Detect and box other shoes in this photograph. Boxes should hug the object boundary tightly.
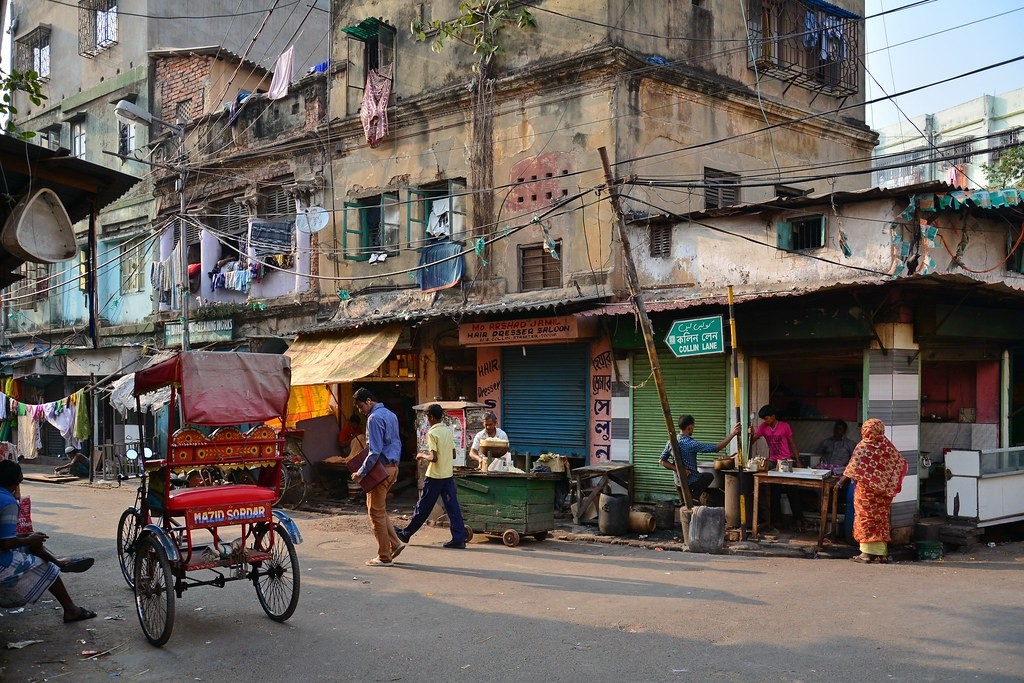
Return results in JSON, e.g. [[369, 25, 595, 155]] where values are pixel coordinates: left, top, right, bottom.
[[442, 539, 467, 548], [393, 524, 409, 543]]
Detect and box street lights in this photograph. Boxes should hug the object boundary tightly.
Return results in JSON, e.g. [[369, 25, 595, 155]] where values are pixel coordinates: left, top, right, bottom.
[[110, 100, 189, 353]]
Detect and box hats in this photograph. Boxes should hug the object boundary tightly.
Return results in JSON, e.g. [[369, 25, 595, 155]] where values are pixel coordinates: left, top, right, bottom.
[[65, 447, 76, 453]]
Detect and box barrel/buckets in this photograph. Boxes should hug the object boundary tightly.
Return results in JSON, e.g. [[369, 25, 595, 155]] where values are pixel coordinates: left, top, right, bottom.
[[628, 512, 656, 534], [598, 492, 629, 535], [348, 480, 365, 500], [915, 540, 944, 560], [655, 500, 675, 530]]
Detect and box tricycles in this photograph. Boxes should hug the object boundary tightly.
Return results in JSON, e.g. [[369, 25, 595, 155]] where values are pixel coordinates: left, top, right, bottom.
[[181, 428, 310, 511], [115, 350, 303, 645]]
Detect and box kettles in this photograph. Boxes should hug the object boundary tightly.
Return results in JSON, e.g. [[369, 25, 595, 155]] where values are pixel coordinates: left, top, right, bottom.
[[744, 456, 767, 472]]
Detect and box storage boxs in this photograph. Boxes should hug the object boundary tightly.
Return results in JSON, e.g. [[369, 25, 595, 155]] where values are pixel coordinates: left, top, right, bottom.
[[532, 457, 566, 472]]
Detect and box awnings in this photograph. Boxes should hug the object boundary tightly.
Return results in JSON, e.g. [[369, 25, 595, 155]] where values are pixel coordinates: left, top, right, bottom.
[[283, 325, 402, 387]]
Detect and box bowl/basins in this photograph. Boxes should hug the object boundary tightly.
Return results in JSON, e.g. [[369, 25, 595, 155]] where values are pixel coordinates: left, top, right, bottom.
[[777, 459, 794, 472], [479, 446, 509, 458]]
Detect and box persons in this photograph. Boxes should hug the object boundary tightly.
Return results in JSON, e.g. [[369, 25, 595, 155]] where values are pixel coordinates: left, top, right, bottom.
[[815, 420, 856, 466], [394, 404, 466, 549], [0, 459, 97, 623], [351, 388, 406, 566], [55, 447, 89, 477], [833, 419, 907, 564], [660, 415, 742, 503], [469, 411, 510, 468], [747, 405, 803, 530]]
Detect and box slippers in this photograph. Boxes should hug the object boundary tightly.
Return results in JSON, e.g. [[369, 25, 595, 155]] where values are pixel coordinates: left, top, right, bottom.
[[365, 558, 395, 567], [62, 605, 99, 623], [849, 556, 871, 563], [872, 560, 882, 563], [61, 555, 94, 572], [390, 543, 405, 557]]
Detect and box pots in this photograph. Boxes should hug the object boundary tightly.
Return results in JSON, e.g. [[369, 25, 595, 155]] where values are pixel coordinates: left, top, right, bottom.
[[696, 465, 725, 488], [919, 451, 931, 478], [713, 452, 737, 470]]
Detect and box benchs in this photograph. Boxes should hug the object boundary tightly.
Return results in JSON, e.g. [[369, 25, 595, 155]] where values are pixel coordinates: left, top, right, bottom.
[[143, 425, 288, 512]]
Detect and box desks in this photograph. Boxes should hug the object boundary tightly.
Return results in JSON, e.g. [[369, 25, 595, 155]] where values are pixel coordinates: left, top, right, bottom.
[[752, 471, 838, 550], [570, 460, 635, 525], [312, 461, 349, 485]]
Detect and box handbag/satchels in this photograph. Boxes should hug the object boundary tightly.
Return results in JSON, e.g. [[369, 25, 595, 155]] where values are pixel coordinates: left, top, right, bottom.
[[348, 446, 389, 494]]
